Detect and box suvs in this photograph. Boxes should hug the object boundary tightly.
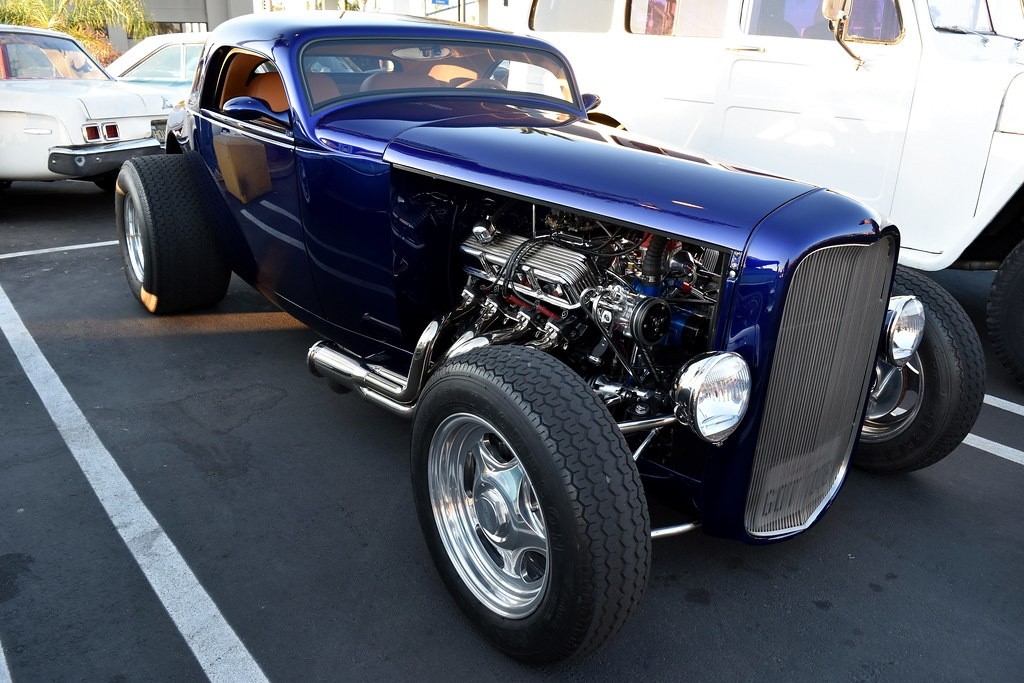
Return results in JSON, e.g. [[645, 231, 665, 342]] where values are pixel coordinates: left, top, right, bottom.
[[495, 0, 1023, 389]]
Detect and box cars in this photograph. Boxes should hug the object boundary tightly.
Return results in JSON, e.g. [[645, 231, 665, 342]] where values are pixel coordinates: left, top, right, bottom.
[[1, 24, 189, 215], [109, 11, 987, 673], [101, 28, 368, 98]]
